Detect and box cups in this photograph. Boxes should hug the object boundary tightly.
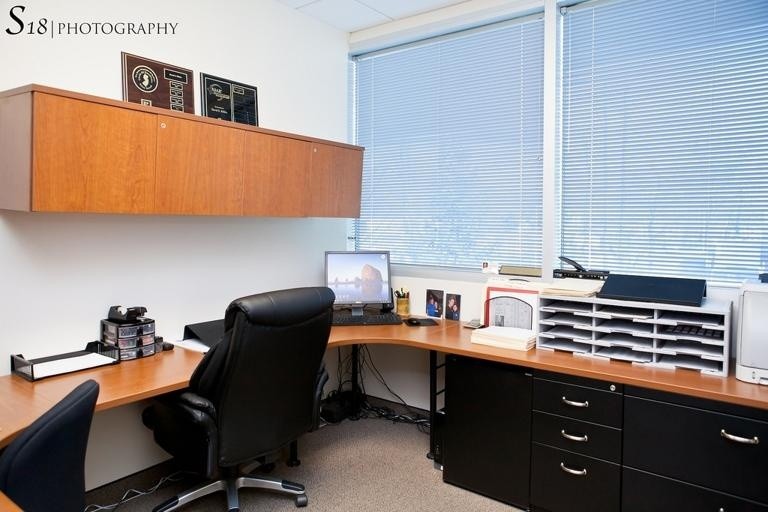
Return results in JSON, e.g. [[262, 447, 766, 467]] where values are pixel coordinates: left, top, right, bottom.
[[396, 298, 409, 314]]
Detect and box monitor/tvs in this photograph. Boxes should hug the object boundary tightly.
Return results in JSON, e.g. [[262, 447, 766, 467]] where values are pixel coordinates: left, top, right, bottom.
[[324, 250, 391, 316]]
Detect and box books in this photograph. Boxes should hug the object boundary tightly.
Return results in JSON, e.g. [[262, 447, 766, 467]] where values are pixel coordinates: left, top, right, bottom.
[[469, 275, 605, 351]]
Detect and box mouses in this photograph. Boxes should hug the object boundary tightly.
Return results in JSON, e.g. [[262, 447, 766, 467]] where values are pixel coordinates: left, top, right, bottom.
[[407, 319, 422, 326]]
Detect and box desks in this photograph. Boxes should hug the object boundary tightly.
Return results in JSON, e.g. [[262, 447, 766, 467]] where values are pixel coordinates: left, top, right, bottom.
[[1, 314, 768, 512]]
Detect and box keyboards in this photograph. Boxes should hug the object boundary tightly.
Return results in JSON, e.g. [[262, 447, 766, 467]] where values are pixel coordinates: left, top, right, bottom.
[[332, 316, 403, 326]]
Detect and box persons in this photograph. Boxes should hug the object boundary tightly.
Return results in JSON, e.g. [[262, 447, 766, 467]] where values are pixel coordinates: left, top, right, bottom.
[[444, 295, 457, 321], [452, 304, 459, 321], [434, 301, 442, 318], [426, 294, 436, 316]]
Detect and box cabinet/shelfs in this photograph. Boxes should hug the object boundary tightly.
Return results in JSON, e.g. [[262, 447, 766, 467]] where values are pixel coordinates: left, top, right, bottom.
[[443, 353, 768, 512], [0, 83, 367, 217], [534, 288, 734, 377]]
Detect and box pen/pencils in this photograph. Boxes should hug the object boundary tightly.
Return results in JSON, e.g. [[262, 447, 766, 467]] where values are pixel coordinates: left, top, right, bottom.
[[394, 287, 409, 298]]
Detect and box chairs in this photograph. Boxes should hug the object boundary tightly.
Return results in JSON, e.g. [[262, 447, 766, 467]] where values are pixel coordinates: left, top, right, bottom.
[[1, 378, 100, 512], [140, 286, 335, 512]]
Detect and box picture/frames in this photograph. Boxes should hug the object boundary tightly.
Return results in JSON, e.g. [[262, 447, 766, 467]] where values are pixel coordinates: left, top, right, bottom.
[[120, 52, 258, 127]]
